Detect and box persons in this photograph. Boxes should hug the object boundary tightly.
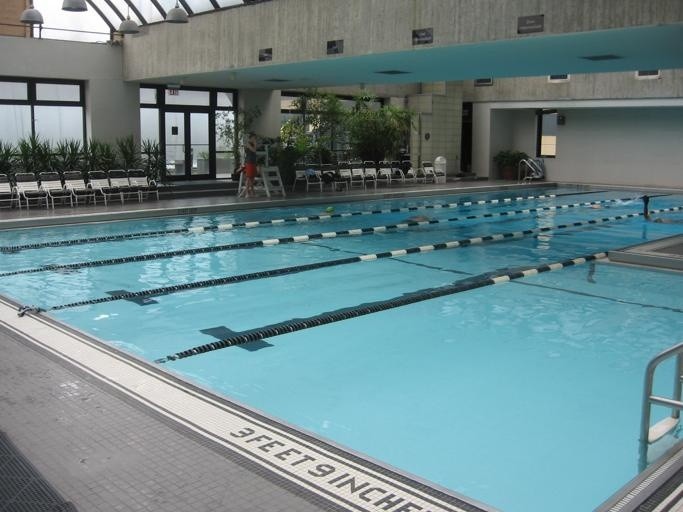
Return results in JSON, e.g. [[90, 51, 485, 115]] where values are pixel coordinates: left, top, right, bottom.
[[245, 134, 259, 198]]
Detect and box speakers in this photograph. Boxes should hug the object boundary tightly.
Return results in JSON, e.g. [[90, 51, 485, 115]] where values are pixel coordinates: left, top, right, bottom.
[[557, 115, 565, 125]]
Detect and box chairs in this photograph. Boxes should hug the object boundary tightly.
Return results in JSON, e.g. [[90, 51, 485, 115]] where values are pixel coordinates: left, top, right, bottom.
[[0, 169, 161, 211], [291, 159, 438, 193]]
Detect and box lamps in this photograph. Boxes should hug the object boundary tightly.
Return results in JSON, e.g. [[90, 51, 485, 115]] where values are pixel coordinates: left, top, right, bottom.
[[18, 1, 189, 34]]
